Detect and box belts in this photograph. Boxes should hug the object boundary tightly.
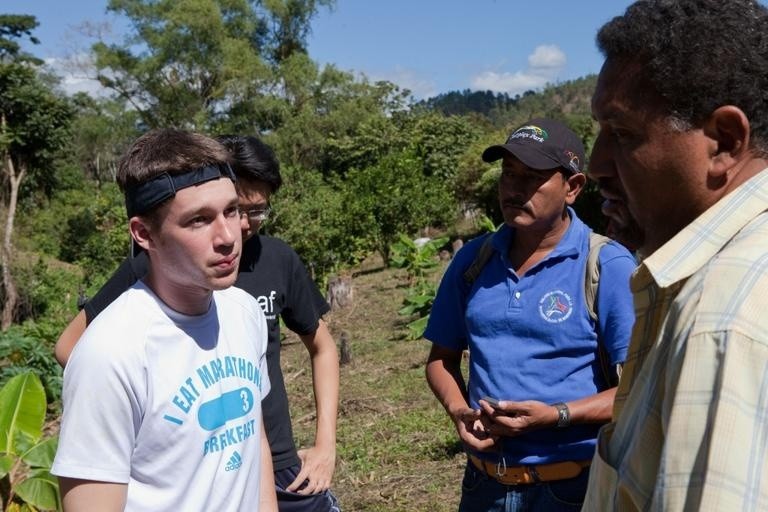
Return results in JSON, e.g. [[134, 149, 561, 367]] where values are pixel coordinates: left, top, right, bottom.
[[467, 454, 592, 485]]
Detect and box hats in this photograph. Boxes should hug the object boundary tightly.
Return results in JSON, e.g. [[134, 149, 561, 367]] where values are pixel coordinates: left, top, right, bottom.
[[482, 116, 586, 176], [126, 158, 237, 260]]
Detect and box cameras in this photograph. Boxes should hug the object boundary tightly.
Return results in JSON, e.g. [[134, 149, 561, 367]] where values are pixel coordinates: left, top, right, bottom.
[[481, 396, 520, 425]]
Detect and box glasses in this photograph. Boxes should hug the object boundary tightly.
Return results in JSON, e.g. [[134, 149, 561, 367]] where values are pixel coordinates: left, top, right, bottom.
[[242, 201, 274, 222]]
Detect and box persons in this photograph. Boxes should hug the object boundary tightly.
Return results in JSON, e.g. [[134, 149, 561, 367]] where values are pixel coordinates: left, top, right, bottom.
[[579, 1, 767, 512], [50, 126, 280, 512], [424, 118, 639, 512], [54, 133, 343, 512]]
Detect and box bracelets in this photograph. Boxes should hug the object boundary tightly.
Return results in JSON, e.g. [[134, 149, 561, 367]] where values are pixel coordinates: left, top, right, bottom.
[[550, 401, 571, 430]]
